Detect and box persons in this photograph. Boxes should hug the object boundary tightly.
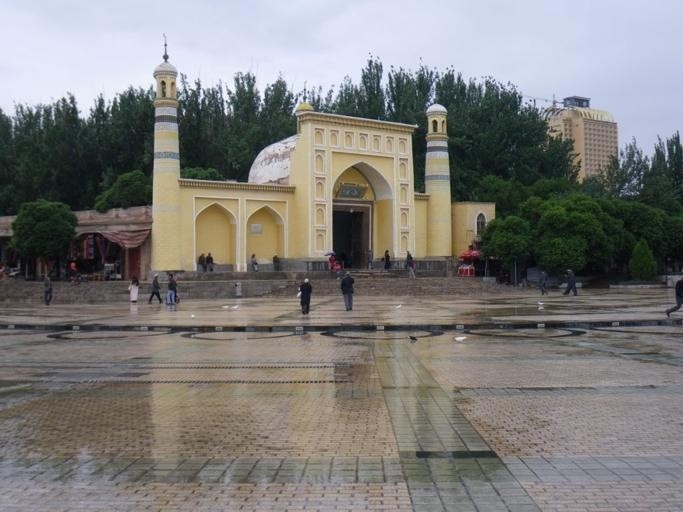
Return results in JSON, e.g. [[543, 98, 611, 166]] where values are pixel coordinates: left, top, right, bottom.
[[537, 269, 548, 295], [299, 278, 311, 314], [328, 254, 336, 272], [196, 253, 205, 273], [127, 275, 139, 303], [407, 257, 415, 280], [332, 261, 343, 279], [166, 273, 176, 305], [43, 274, 52, 306], [560, 268, 577, 295], [664, 276, 682, 318], [340, 271, 354, 311], [148, 273, 162, 304], [383, 249, 391, 270], [205, 253, 212, 272], [272, 253, 280, 271], [250, 254, 257, 272], [404, 250, 412, 269]]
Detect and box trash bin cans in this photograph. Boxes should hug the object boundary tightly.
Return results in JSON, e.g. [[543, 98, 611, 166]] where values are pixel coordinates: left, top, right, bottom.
[[666, 275, 673, 288], [234, 283, 242, 297]]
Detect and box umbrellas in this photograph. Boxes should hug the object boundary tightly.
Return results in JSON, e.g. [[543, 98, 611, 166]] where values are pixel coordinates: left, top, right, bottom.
[[470, 247, 497, 277], [323, 251, 336, 256], [457, 248, 476, 266]]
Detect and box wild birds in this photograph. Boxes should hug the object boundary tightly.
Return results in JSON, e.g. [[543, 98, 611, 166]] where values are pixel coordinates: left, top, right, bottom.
[[222, 305, 229, 309], [191, 314, 195, 319], [396, 304, 402, 309], [537, 300, 545, 305], [409, 334, 418, 343], [537, 306, 544, 311], [232, 305, 239, 310], [452, 336, 468, 343]]
